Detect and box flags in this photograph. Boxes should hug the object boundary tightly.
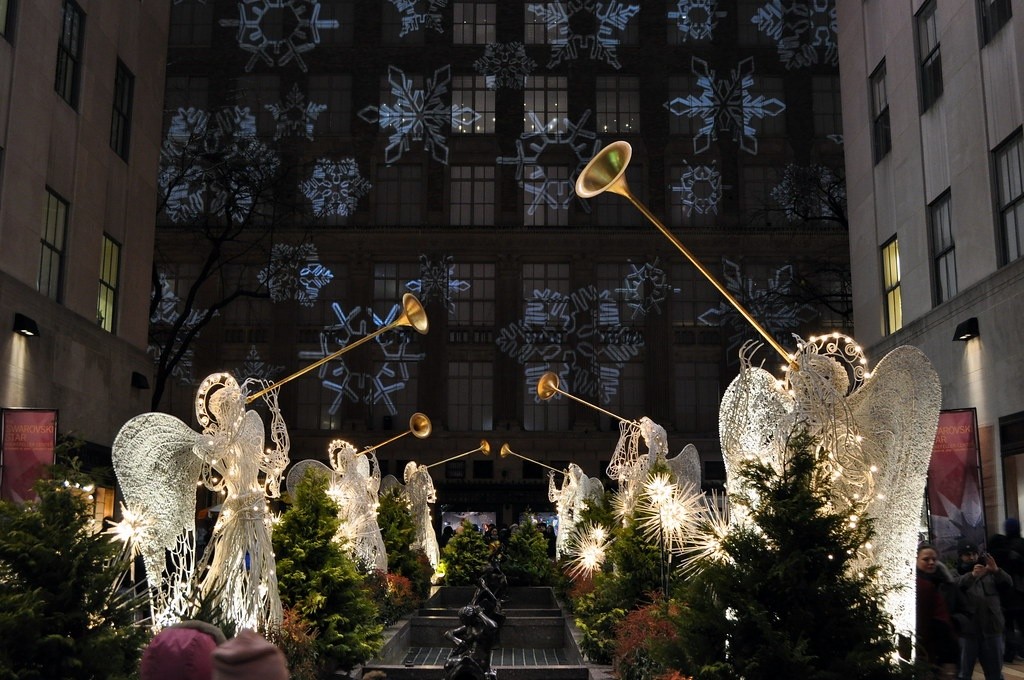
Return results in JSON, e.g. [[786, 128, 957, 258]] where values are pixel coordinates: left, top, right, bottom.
[[2, 410, 57, 513], [926, 412, 987, 561]]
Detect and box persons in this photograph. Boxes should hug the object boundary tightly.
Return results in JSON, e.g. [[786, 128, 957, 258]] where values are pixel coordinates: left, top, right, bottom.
[[916, 518, 1024, 680], [91, 517, 115, 539], [439, 523, 557, 559]]
